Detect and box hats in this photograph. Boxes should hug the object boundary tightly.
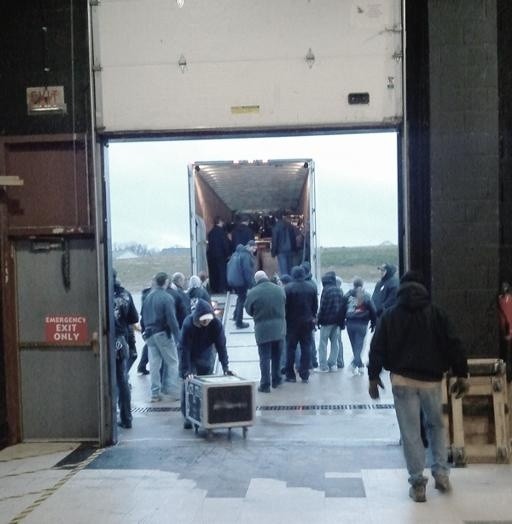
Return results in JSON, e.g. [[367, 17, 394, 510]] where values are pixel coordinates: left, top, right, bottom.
[[247, 240, 257, 247], [241, 214, 249, 221], [276, 209, 282, 219], [379, 264, 386, 271]]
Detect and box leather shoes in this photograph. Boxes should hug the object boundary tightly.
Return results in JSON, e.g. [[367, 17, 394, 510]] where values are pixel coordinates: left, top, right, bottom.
[[258, 387, 269, 392], [183, 417, 193, 429], [137, 369, 150, 375], [272, 378, 282, 387], [236, 322, 249, 329]]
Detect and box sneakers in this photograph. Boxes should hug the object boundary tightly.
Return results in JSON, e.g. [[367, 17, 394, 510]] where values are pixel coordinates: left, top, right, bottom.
[[408, 483, 426, 501], [435, 478, 450, 492], [170, 397, 180, 402], [151, 396, 162, 403], [357, 367, 364, 374], [281, 367, 286, 374], [313, 364, 338, 373], [117, 421, 132, 428], [286, 378, 295, 382], [302, 379, 308, 384], [347, 365, 356, 375]]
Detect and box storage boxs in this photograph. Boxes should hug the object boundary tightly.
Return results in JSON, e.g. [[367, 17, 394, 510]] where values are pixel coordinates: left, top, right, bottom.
[[185, 374, 257, 429]]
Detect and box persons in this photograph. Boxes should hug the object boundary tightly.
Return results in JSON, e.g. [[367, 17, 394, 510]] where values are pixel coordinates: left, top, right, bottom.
[[186, 275, 209, 303], [207, 217, 229, 293], [314, 271, 343, 372], [343, 279, 377, 378], [177, 299, 237, 430], [371, 263, 399, 327], [245, 269, 286, 394], [270, 272, 281, 286], [335, 277, 344, 369], [141, 272, 181, 404], [126, 324, 137, 389], [271, 213, 295, 275], [171, 273, 191, 314], [280, 275, 301, 374], [498, 281, 511, 384], [166, 276, 188, 330], [198, 271, 212, 297], [112, 268, 139, 429], [226, 241, 258, 329], [232, 216, 255, 253], [137, 287, 152, 375], [367, 271, 469, 503], [284, 267, 318, 385], [300, 262, 318, 368]]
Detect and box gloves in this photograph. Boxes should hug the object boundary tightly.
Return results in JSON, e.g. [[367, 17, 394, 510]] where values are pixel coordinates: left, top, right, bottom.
[[271, 249, 276, 256], [450, 376, 470, 399], [368, 376, 383, 400], [184, 371, 195, 380], [225, 368, 239, 376], [368, 324, 375, 333]]
